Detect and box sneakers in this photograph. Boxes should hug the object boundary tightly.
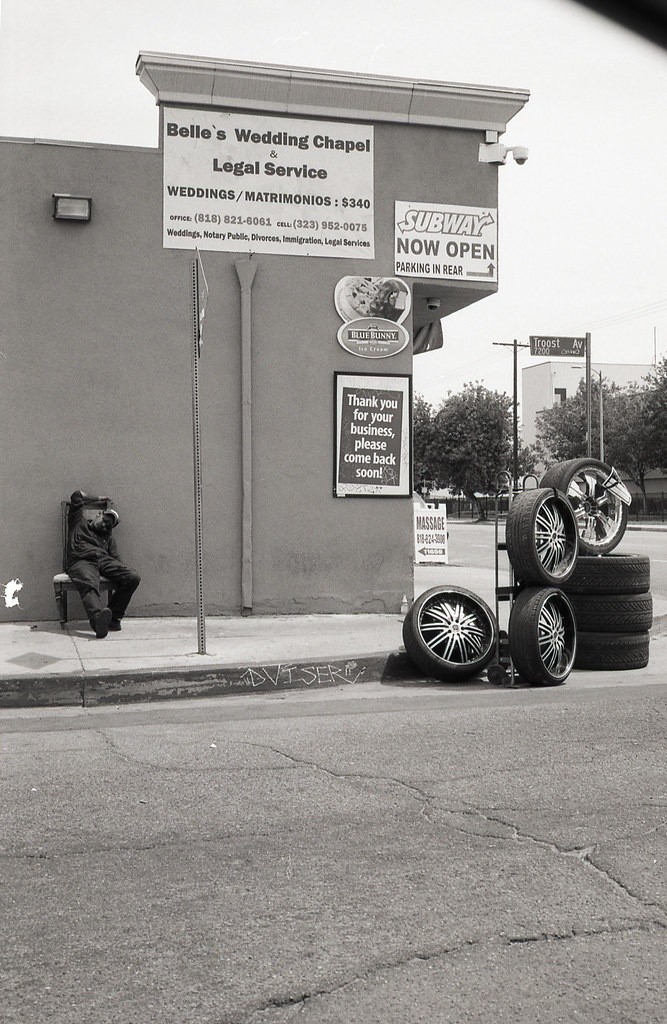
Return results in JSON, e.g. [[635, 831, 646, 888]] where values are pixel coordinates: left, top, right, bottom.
[[91, 608, 121, 638]]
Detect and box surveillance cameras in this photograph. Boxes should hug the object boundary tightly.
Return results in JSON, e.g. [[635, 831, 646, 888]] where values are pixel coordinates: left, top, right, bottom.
[[513, 145, 527, 165], [427, 298, 440, 310]]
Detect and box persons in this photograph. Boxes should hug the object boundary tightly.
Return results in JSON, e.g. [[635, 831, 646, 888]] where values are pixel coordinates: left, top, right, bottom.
[[63, 490, 141, 637]]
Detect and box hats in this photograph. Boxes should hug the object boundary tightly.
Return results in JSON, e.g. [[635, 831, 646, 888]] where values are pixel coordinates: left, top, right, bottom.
[[103, 508, 119, 526]]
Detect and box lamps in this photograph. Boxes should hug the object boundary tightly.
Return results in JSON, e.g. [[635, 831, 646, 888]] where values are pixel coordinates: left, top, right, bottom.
[[50, 191, 92, 221]]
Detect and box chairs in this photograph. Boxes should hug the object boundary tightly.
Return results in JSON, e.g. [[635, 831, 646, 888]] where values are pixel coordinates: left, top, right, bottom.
[[53, 500, 114, 630]]
